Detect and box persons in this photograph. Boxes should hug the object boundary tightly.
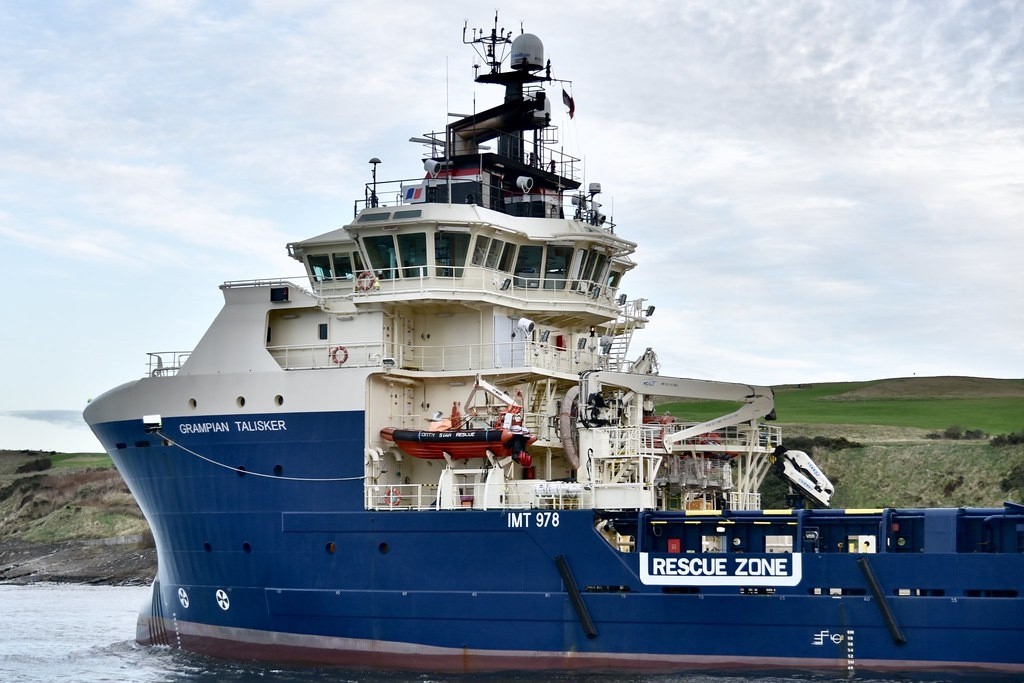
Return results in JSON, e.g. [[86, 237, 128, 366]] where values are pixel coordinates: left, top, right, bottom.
[[861, 541, 871, 553], [277, 288, 287, 301]]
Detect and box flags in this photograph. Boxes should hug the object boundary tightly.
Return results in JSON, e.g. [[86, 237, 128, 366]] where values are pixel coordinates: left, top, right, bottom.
[[563, 89, 574, 119]]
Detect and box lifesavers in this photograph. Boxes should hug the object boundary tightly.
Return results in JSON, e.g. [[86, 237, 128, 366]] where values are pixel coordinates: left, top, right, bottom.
[[358, 272, 374, 290], [385, 488, 400, 506], [332, 346, 348, 363]]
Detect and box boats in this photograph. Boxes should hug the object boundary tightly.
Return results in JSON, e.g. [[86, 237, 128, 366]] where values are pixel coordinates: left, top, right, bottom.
[[379, 404, 536, 466], [77, 7, 1024, 679]]
